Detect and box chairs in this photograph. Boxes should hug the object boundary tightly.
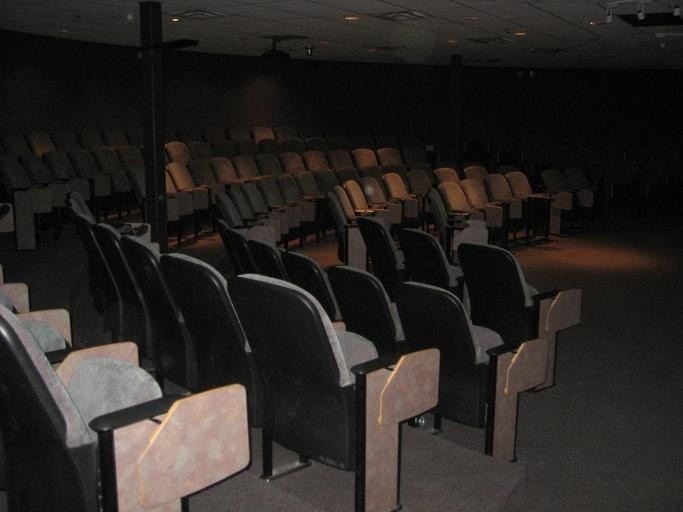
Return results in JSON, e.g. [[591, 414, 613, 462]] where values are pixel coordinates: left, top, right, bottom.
[[0, 124, 594, 357], [400, 282, 549, 461], [2, 192, 263, 512], [237, 273, 440, 512], [458, 241, 583, 392]]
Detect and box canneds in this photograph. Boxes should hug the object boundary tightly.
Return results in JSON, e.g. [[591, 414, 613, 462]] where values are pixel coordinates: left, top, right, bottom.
[[409, 416, 425, 428]]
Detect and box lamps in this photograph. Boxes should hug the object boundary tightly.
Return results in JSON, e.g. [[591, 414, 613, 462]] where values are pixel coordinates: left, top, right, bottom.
[[599, 0, 682, 23]]
